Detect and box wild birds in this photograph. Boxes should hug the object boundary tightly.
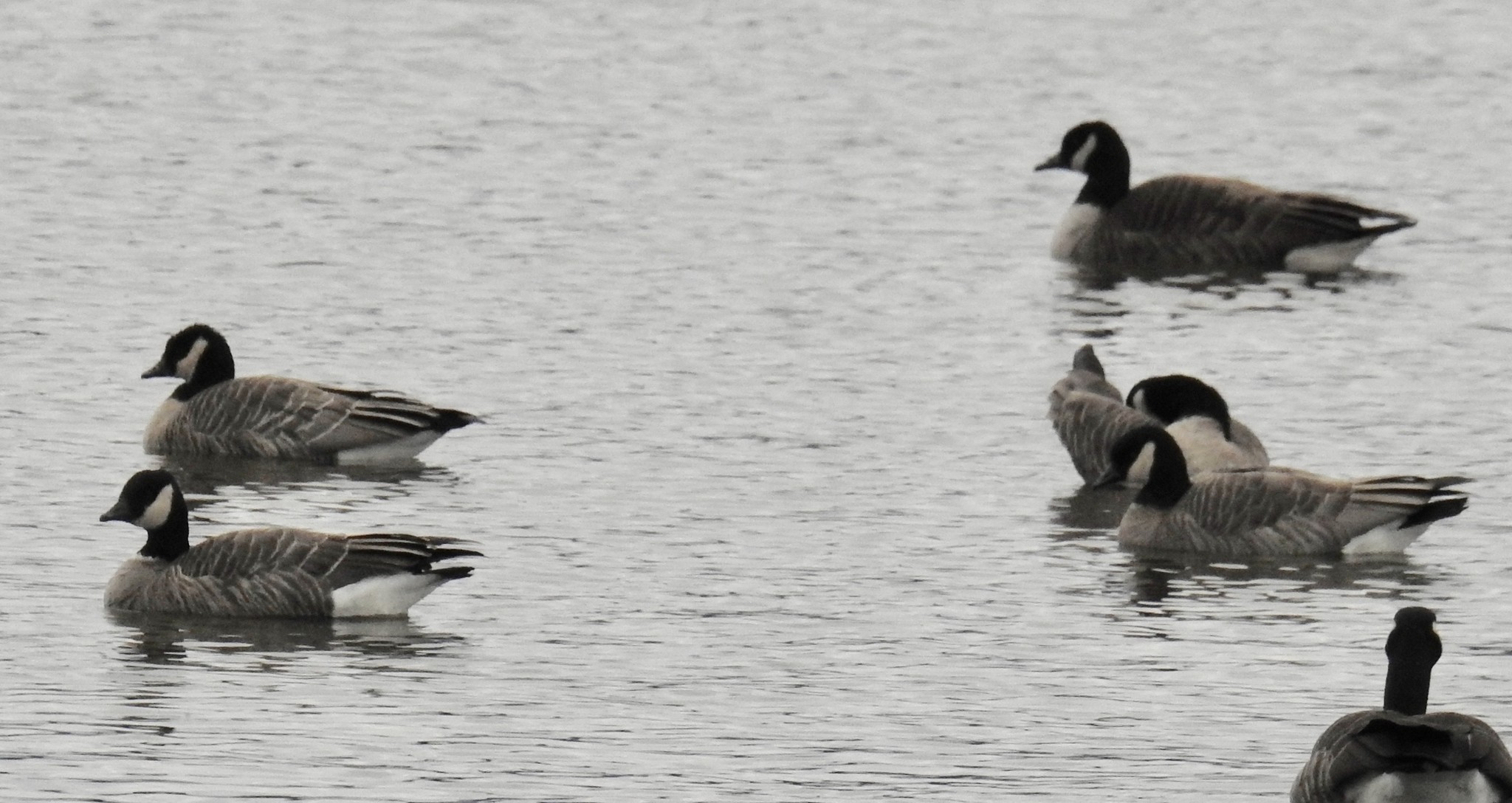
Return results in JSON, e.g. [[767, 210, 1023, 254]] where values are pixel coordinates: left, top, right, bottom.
[[1292, 606, 1512, 803], [1037, 121, 1418, 274], [1048, 343, 1475, 558], [142, 323, 487, 471], [99, 468, 488, 620]]
